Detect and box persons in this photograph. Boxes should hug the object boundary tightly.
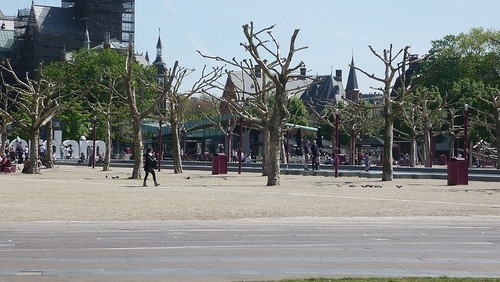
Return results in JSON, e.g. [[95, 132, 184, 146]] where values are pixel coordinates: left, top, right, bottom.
[[78, 152, 86, 163], [180, 147, 211, 161], [96, 153, 105, 165], [230, 148, 257, 164], [0, 141, 73, 167], [303, 139, 312, 171], [143, 147, 160, 187], [354, 149, 373, 171], [310, 140, 320, 171]]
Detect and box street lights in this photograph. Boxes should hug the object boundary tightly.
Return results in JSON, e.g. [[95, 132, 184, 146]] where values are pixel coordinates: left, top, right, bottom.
[[463, 104, 468, 167], [92, 115, 97, 168], [334, 109, 339, 176], [239, 110, 243, 174], [351, 125, 355, 165], [429, 124, 433, 167]]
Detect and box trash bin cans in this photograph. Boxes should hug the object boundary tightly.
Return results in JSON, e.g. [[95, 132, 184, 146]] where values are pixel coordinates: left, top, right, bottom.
[[446, 157, 468, 186], [211, 152, 228, 175]]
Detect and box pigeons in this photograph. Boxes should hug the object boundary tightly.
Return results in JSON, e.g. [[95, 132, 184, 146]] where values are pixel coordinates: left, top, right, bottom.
[[184, 176, 191, 180], [112, 175, 119, 179], [349, 184, 384, 189], [336, 184, 342, 187], [17, 166, 21, 170], [105, 175, 108, 178], [395, 184, 403, 189]]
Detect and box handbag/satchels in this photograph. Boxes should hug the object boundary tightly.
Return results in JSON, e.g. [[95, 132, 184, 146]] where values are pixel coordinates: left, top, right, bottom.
[[151, 160, 158, 169]]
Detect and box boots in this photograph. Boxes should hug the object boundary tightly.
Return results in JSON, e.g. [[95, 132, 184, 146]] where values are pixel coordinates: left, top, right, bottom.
[[154, 181, 160, 186], [143, 182, 147, 186]]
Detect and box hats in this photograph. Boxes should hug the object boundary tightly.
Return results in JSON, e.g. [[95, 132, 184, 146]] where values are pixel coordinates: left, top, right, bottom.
[[147, 147, 153, 150]]
